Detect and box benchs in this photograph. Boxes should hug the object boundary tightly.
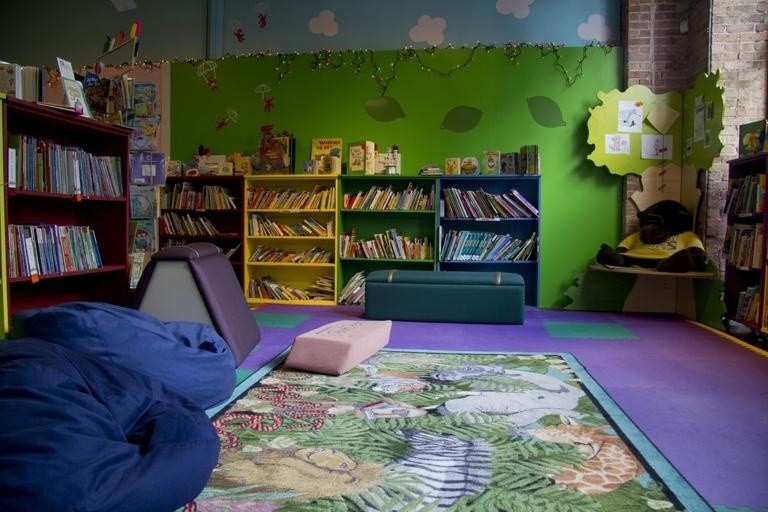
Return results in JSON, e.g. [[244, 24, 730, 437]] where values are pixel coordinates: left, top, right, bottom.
[[362, 270, 524, 325]]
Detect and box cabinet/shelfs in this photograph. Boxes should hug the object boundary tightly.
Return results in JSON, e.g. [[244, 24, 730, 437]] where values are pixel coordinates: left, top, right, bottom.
[[2, 94, 133, 334], [722, 151, 767, 351]]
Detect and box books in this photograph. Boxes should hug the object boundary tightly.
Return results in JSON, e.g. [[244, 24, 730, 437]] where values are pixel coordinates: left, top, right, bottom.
[[311, 137, 402, 176], [339, 184, 435, 302], [0, 55, 163, 291], [439, 188, 538, 263], [165, 133, 296, 176], [418, 144, 539, 177], [247, 184, 335, 301], [164, 181, 241, 260], [720, 116, 767, 338]]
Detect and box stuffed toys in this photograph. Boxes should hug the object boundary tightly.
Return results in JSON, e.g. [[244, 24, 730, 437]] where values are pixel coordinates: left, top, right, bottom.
[[594, 199, 708, 274]]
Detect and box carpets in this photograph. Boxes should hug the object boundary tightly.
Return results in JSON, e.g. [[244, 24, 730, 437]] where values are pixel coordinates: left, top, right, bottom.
[[167, 343, 716, 512]]
